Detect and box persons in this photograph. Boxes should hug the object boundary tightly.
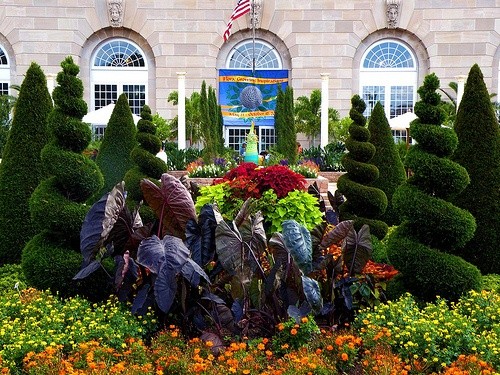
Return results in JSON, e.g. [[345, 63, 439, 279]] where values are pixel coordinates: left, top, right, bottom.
[[297, 141, 305, 154]]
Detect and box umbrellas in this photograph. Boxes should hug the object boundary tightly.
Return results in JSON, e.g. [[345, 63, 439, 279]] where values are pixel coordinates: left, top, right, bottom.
[[83, 101, 146, 132], [387, 109, 455, 133]]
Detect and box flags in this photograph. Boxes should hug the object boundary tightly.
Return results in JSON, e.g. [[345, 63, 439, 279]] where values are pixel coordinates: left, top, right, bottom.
[[222, 0, 252, 42], [217, 68, 290, 126]]
